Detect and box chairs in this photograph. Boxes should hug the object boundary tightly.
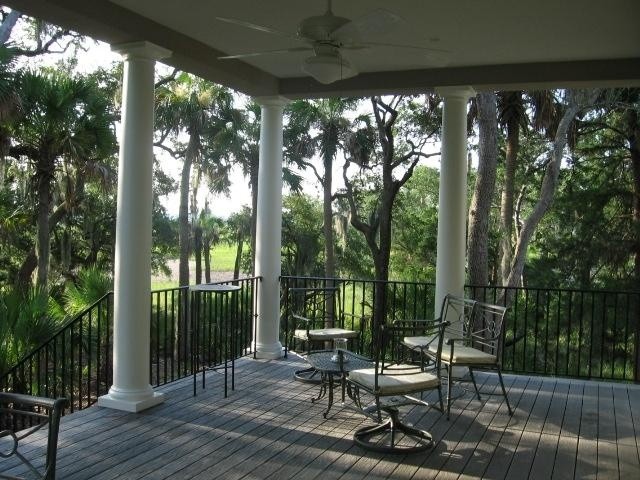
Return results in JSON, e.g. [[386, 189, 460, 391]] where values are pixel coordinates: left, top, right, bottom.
[[284, 285, 515, 454]]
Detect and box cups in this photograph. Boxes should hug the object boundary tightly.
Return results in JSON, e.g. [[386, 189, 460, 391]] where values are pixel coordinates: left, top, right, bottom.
[[331, 338, 350, 363]]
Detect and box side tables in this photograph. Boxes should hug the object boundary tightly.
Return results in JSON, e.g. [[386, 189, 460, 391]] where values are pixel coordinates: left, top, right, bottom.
[[189, 284, 240, 399]]
